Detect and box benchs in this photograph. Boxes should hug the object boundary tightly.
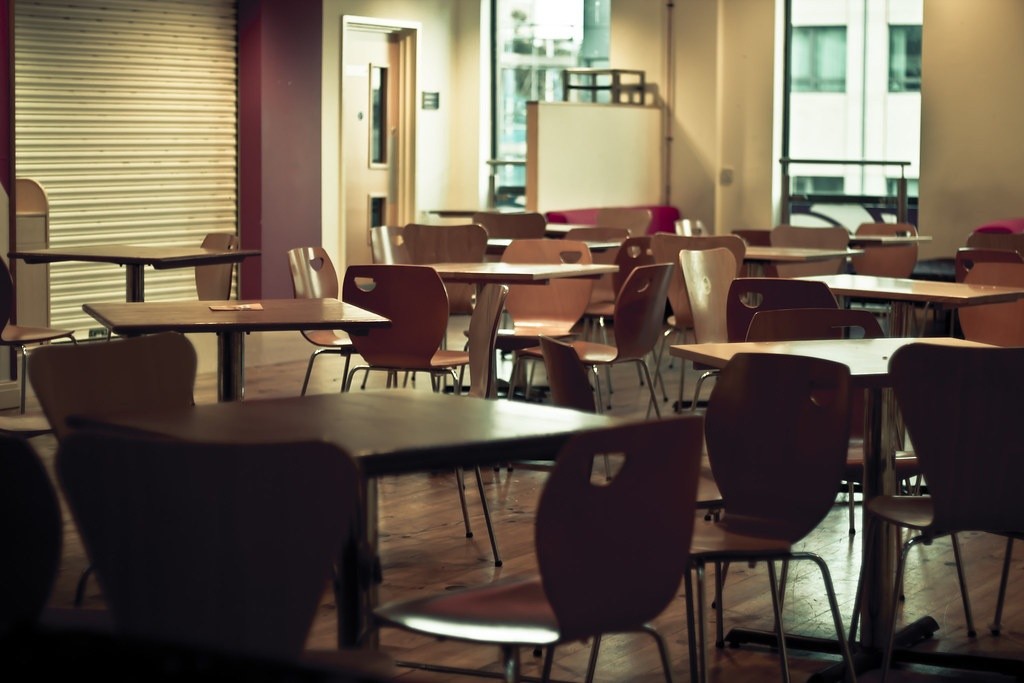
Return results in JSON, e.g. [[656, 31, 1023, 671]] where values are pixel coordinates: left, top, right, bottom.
[[546, 204, 682, 239]]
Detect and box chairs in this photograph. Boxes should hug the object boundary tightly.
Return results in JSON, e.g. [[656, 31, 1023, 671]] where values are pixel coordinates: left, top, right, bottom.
[[0, 205, 1024, 683]]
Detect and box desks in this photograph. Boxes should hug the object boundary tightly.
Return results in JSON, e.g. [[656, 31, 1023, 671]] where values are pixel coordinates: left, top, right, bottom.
[[666, 335, 1024, 683], [8, 242, 264, 304], [83, 297, 393, 404], [838, 234, 934, 273], [738, 246, 866, 305], [98, 387, 628, 683], [419, 262, 620, 474], [791, 271, 1024, 540], [482, 238, 621, 266]]
